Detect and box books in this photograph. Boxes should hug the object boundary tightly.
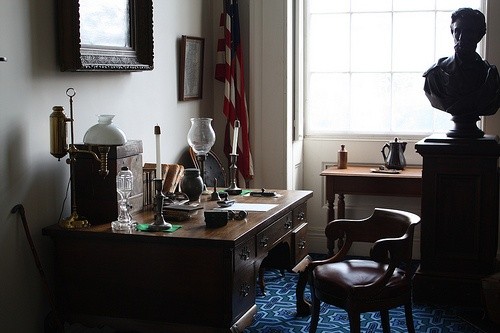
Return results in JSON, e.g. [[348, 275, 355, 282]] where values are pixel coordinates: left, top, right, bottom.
[[143, 163, 185, 206]]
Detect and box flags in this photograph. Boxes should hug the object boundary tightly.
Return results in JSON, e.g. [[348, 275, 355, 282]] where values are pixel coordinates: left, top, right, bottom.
[[215, 1, 255, 182]]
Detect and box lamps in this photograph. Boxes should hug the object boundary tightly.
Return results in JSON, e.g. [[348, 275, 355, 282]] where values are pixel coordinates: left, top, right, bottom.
[[35, 88, 127, 228]]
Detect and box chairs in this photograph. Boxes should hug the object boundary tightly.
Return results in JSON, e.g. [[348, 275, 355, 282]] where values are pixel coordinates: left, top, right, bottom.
[[189, 146, 228, 188], [294, 207, 422, 333]]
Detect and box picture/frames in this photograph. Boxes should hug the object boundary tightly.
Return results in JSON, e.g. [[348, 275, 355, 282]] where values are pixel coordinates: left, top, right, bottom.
[[58, 1, 155, 72], [176, 34, 207, 101]]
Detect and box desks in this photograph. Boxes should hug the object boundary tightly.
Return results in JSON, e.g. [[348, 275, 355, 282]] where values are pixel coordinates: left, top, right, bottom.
[[320, 165, 423, 257], [42, 187, 313, 333]]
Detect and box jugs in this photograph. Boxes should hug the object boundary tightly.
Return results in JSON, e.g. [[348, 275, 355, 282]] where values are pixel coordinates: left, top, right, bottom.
[[381, 138, 407, 170]]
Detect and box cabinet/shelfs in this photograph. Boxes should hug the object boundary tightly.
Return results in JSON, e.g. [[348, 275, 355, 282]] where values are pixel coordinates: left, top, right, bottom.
[[415, 135, 500, 280]]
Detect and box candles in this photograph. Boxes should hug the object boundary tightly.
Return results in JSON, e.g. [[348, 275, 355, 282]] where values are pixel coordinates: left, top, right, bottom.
[[231, 118, 240, 154], [156, 120, 161, 179]]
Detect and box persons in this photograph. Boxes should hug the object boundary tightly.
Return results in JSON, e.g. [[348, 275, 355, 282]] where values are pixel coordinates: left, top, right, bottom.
[[423, 8, 500, 138]]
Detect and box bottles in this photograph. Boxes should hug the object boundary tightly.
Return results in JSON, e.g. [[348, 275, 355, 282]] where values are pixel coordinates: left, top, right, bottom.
[[337, 145, 348, 169], [181, 168, 204, 202]]
